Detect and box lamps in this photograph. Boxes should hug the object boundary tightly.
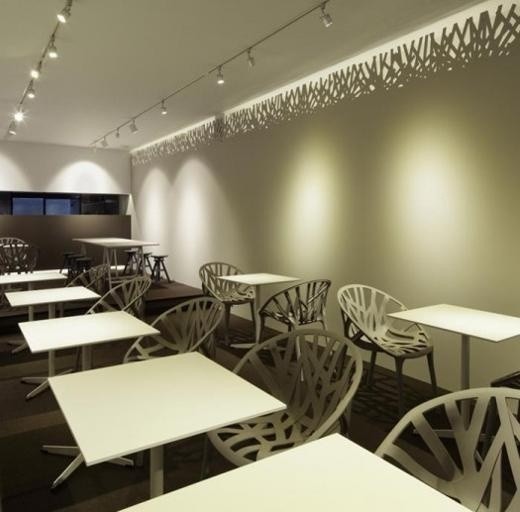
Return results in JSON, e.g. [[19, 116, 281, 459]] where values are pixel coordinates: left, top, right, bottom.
[[216, 67, 225, 86], [130, 122, 139, 137], [14, 110, 26, 127], [8, 121, 18, 137], [26, 85, 36, 99], [56, 8, 72, 26], [159, 100, 170, 117], [115, 130, 121, 140], [47, 40, 60, 60], [31, 66, 43, 80], [245, 49, 257, 68], [320, 7, 333, 28], [101, 138, 108, 151]]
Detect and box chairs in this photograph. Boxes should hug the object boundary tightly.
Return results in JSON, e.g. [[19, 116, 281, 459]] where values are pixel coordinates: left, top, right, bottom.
[[199, 262, 255, 328], [202, 329, 363, 476], [374, 387, 520, 512], [0, 237, 39, 304], [481, 371, 517, 463], [123, 297, 225, 466], [59, 264, 109, 316], [336, 284, 437, 411], [257, 280, 337, 381], [73, 277, 151, 369]]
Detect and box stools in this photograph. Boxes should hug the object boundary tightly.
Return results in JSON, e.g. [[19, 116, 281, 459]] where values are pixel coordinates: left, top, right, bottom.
[[150, 254, 173, 284], [62, 252, 74, 269], [138, 252, 152, 274], [122, 250, 138, 274], [68, 254, 84, 276], [75, 258, 92, 274]]
